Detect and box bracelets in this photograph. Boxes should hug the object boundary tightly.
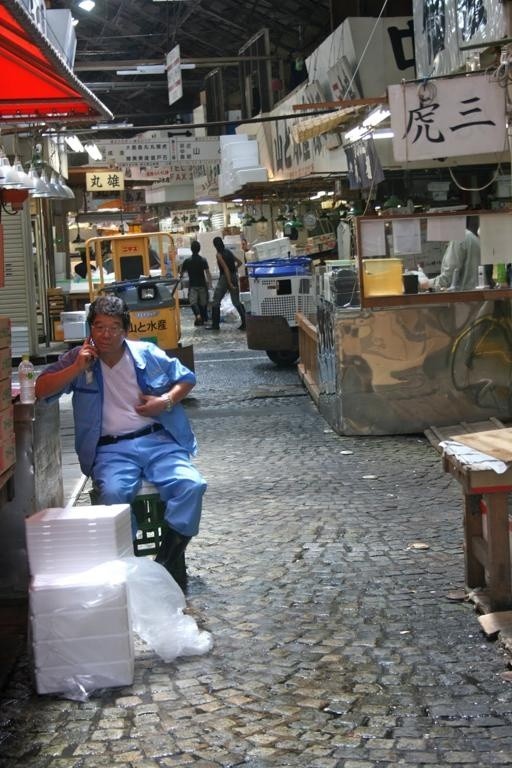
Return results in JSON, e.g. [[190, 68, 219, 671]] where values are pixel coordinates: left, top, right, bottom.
[[162, 393, 174, 413]]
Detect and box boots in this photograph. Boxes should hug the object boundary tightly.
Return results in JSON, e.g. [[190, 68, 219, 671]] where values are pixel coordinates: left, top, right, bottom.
[[191, 302, 208, 326], [235, 304, 247, 329], [152, 527, 192, 570], [205, 305, 220, 330]]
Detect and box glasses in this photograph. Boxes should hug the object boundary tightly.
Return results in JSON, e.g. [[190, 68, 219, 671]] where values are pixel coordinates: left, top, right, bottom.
[[91, 323, 126, 336]]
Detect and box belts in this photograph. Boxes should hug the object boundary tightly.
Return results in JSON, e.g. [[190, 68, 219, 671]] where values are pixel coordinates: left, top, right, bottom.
[[118, 422, 163, 440]]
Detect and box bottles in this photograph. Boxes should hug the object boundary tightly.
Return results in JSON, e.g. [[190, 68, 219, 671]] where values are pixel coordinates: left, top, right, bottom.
[[18, 352, 37, 406]]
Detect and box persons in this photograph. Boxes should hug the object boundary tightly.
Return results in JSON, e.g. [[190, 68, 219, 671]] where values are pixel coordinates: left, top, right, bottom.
[[205, 236, 246, 330], [32, 295, 206, 570], [419, 228, 481, 295], [76, 251, 96, 279], [179, 241, 213, 324]]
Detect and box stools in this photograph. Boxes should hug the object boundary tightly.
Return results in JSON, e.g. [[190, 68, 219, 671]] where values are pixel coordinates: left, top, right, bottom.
[[90, 480, 166, 560]]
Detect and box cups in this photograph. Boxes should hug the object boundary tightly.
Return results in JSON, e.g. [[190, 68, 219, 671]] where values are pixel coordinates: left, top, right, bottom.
[[401, 274, 420, 294]]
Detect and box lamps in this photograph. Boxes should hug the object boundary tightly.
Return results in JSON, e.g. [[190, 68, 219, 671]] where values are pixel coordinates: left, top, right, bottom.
[[0, 105, 77, 201]]
